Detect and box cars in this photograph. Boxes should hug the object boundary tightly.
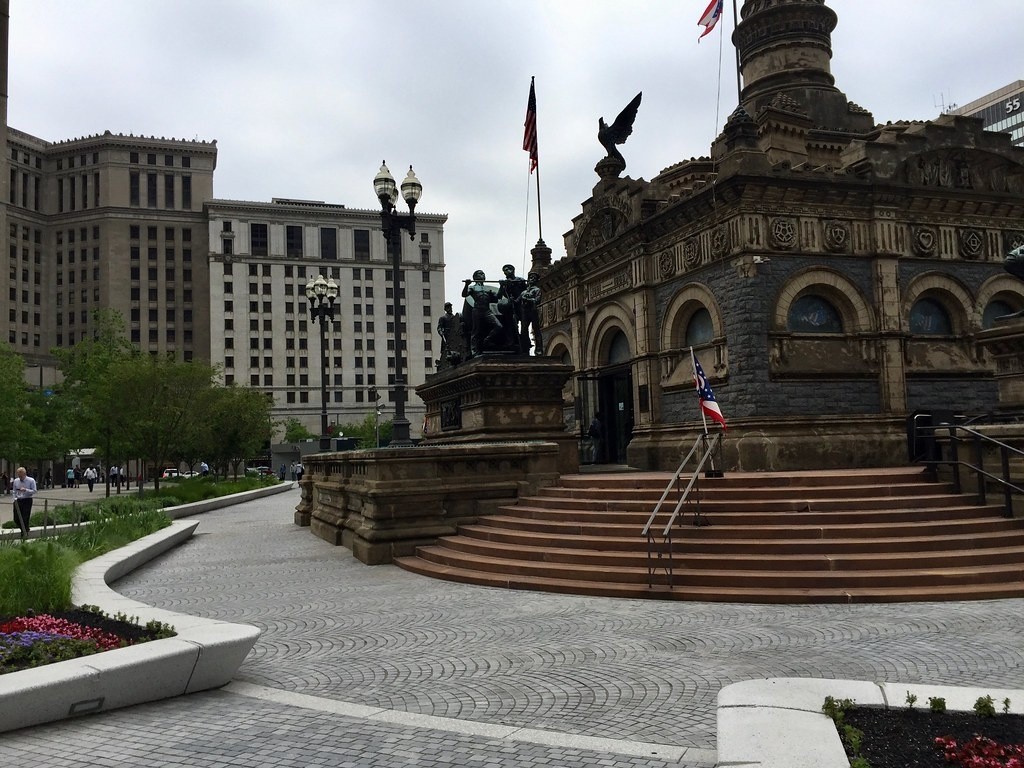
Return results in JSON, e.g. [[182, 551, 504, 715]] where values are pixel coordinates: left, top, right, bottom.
[[256, 466, 275, 477], [163, 469, 183, 479], [184, 470, 201, 479]]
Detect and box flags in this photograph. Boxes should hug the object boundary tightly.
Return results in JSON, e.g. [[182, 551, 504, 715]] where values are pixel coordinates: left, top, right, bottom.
[[696, 0, 723, 42], [694, 354, 726, 430], [522, 81, 539, 174]]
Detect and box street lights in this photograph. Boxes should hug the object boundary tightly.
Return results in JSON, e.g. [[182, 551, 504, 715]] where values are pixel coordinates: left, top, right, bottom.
[[305, 272, 339, 455], [373, 160, 423, 448]]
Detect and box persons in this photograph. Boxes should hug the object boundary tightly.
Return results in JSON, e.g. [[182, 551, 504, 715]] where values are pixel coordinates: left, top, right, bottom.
[[0, 465, 172, 491], [290, 461, 296, 481], [297, 461, 305, 481], [200, 462, 208, 477], [512, 271, 544, 357], [437, 302, 455, 358], [267, 468, 272, 474], [461, 269, 503, 357], [119, 466, 125, 486], [496, 264, 527, 301], [84, 464, 97, 492], [72, 465, 82, 488], [65, 467, 74, 488], [280, 463, 286, 480], [109, 464, 118, 487], [12, 467, 37, 539]]
